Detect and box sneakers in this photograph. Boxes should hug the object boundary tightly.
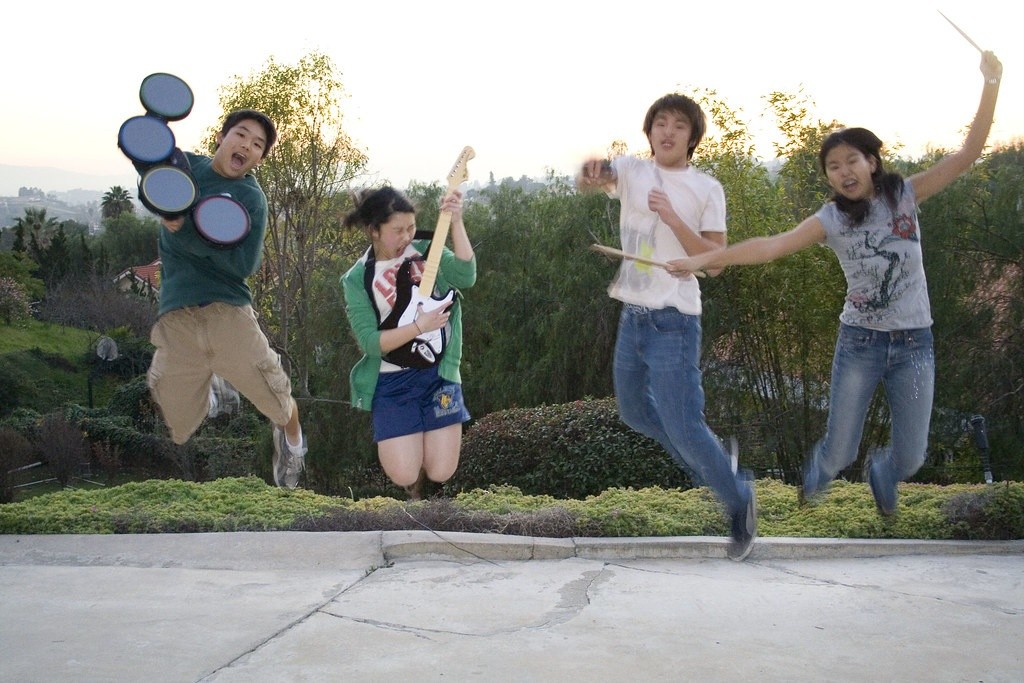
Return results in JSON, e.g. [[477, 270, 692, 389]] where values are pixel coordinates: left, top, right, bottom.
[[271, 424, 308, 495], [719, 436, 740, 477], [210, 373, 242, 419], [726, 486, 760, 563]]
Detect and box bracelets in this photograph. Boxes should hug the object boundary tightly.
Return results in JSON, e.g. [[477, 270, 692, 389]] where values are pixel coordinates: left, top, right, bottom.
[[984, 78, 998, 83], [413, 322, 423, 335]]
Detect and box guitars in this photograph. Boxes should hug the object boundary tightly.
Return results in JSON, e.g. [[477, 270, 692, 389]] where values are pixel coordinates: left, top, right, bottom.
[[375, 145, 477, 371]]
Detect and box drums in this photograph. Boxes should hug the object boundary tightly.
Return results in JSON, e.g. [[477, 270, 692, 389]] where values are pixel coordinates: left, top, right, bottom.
[[190, 195, 252, 250], [137, 164, 202, 221], [139, 72, 195, 122], [117, 116, 176, 164]]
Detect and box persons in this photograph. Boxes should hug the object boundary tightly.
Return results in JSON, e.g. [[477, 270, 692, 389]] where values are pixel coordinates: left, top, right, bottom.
[[146, 110, 307, 495], [339, 186, 478, 486], [584, 92, 758, 560], [662, 49, 1004, 520]]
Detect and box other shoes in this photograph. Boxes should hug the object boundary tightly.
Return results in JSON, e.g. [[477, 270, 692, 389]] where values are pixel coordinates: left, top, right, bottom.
[[802, 436, 834, 501], [867, 462, 899, 518]]
